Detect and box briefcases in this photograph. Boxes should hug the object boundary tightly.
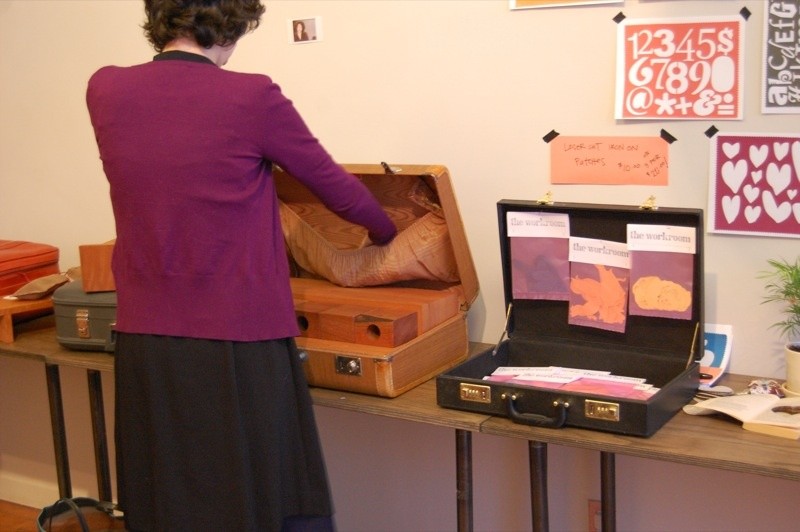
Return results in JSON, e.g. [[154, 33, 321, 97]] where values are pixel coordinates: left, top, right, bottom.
[[290, 156, 480, 398], [0, 238, 59, 299], [436, 191, 706, 439], [50, 276, 120, 352]]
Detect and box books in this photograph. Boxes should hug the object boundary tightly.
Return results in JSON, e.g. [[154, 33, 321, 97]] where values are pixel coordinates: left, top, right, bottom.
[[683, 394, 800, 440]]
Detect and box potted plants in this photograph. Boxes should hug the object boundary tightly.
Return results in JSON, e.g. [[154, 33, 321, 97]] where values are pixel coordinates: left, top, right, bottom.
[[762, 258, 800, 401]]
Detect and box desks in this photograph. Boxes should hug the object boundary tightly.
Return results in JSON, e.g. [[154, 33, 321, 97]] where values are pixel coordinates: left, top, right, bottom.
[[0, 313, 800, 532]]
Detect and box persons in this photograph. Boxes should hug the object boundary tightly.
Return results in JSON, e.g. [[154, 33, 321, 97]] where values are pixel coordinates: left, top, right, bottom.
[[294, 22, 308, 41], [86, 0, 399, 532]]
